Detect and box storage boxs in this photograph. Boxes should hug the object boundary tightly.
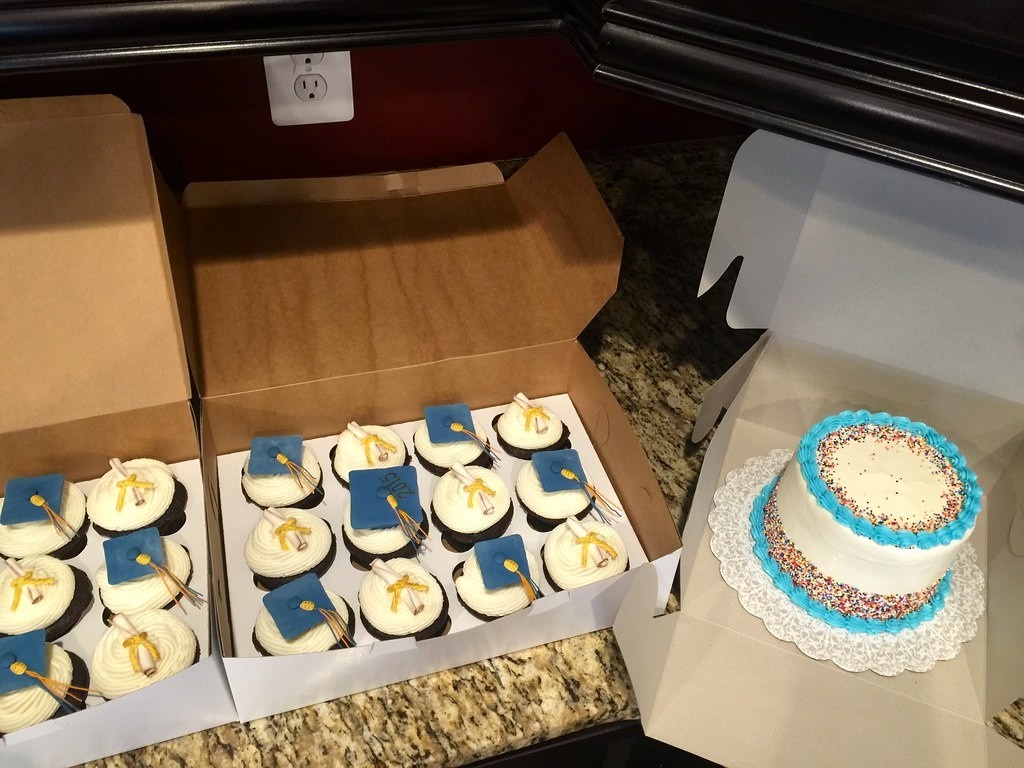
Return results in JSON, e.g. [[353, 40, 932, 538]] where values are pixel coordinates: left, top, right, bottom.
[[0, 93, 1024, 768]]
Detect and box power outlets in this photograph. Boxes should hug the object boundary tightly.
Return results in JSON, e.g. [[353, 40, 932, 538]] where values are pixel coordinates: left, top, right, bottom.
[[262, 51, 354, 126]]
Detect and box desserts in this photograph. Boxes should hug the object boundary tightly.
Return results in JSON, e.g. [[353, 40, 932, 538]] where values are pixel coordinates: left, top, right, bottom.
[[0, 455, 202, 734], [241, 391, 631, 656]]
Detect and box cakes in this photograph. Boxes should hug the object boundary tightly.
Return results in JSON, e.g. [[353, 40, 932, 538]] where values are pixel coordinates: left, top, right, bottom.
[[748, 410, 983, 634]]
